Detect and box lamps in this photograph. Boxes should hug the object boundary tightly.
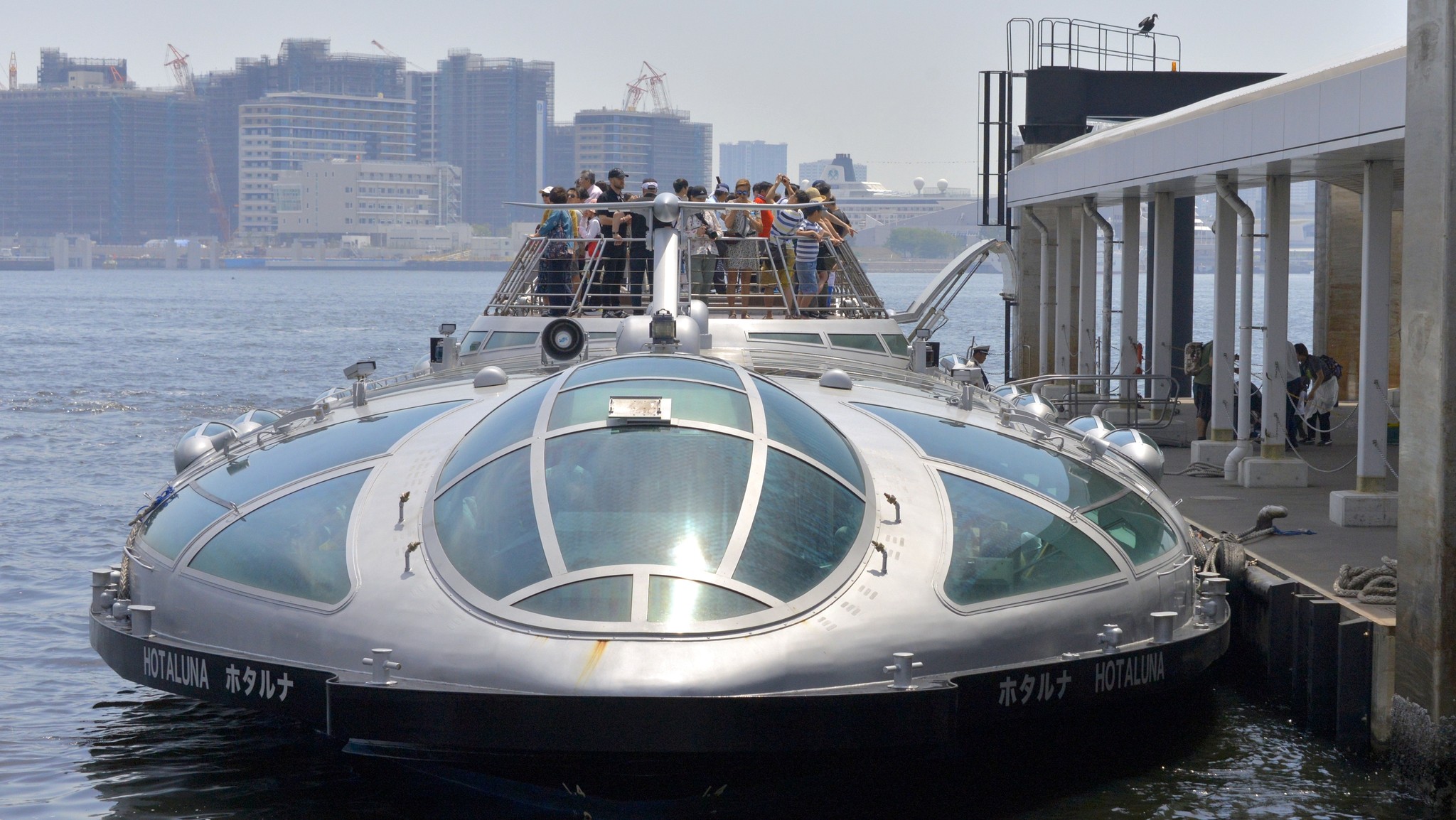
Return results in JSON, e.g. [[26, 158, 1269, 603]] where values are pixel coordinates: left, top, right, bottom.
[[209, 429, 238, 460], [344, 361, 376, 383], [915, 328, 933, 341], [1080, 434, 1110, 465], [439, 324, 456, 337], [951, 368, 981, 385]]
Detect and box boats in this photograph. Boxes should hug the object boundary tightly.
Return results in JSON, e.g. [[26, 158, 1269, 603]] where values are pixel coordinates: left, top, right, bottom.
[[90, 194, 1232, 724]]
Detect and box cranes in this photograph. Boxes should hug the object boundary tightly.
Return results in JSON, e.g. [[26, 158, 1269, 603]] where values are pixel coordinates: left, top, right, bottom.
[[624, 61, 674, 115]]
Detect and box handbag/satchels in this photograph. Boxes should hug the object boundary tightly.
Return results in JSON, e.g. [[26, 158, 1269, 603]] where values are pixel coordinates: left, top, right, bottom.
[[715, 237, 727, 255], [542, 225, 568, 258], [585, 234, 602, 257], [724, 231, 739, 244], [763, 243, 787, 270]]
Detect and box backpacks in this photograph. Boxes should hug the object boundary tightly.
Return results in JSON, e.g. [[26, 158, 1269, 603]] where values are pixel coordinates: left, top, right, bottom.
[[1183, 341, 1209, 376], [1308, 354, 1342, 380]]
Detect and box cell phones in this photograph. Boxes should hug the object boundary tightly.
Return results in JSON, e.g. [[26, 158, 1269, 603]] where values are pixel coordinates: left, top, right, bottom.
[[780, 176, 786, 182]]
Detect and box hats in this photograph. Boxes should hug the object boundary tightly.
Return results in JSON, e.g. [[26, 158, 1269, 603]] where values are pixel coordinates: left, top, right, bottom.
[[806, 187, 826, 203], [717, 183, 729, 188], [971, 345, 990, 355], [716, 186, 728, 192], [806, 200, 828, 216], [539, 186, 554, 193], [737, 215, 750, 237], [588, 199, 597, 213], [812, 179, 826, 187], [608, 168, 629, 179], [641, 179, 657, 189], [692, 185, 709, 199]]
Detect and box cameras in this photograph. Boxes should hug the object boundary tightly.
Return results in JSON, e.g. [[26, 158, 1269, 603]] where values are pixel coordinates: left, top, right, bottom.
[[703, 225, 717, 239]]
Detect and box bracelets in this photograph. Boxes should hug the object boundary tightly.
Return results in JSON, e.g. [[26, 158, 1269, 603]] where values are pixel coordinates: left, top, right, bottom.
[[612, 232, 618, 234], [623, 217, 626, 222], [749, 218, 754, 223]]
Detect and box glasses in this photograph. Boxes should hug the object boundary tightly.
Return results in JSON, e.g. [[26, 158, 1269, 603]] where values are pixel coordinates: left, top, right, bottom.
[[736, 190, 749, 195], [829, 194, 833, 199], [567, 193, 575, 198], [791, 192, 796, 197]]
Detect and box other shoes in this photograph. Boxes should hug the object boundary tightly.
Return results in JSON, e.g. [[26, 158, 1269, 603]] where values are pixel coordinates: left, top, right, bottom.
[[728, 313, 737, 319], [741, 313, 754, 319], [538, 300, 646, 319], [1300, 438, 1315, 443], [761, 309, 836, 320], [1320, 439, 1333, 445]]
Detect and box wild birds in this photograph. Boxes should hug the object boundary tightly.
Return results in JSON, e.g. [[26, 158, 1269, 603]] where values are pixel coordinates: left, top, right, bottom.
[[1134, 13, 1159, 38]]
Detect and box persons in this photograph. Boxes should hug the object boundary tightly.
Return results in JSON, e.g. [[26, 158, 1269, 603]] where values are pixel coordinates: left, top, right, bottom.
[[575, 168, 858, 318], [687, 186, 724, 305], [1286, 340, 1301, 451], [530, 187, 581, 317], [1294, 342, 1340, 446], [1192, 340, 1212, 439]]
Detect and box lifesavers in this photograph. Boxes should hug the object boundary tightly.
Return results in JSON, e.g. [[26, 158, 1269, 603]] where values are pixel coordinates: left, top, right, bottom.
[[1136, 343, 1143, 375]]
